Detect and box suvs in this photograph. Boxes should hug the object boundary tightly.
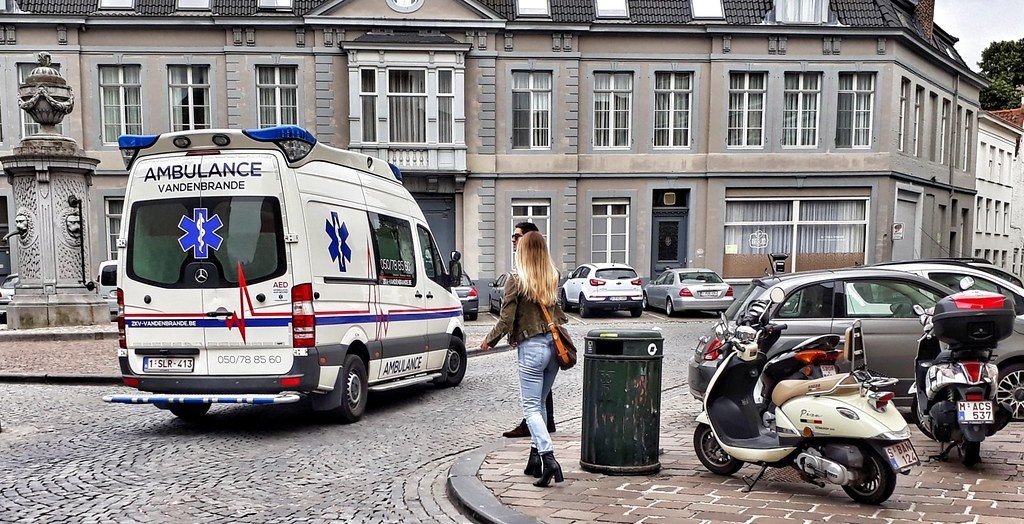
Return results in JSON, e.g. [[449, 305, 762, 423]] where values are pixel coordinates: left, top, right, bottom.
[[561, 263, 643, 318]]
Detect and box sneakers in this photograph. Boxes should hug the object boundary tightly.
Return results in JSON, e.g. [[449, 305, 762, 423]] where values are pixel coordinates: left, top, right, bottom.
[[547, 424, 556, 432], [503, 419, 531, 438]]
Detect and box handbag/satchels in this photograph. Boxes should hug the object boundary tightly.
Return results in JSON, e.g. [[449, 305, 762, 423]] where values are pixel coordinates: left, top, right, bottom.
[[552, 326, 577, 370]]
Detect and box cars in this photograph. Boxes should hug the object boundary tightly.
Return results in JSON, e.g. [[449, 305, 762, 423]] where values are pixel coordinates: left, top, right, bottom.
[[0, 273, 18, 315], [452, 272, 479, 320], [688, 256, 1024, 423], [488, 272, 517, 316], [642, 268, 735, 316]]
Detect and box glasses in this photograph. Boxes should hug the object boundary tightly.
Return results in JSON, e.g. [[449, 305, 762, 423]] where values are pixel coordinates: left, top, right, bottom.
[[512, 234, 523, 241]]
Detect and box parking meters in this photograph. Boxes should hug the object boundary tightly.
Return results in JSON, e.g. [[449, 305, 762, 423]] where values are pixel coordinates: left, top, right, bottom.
[[768, 252, 787, 275]]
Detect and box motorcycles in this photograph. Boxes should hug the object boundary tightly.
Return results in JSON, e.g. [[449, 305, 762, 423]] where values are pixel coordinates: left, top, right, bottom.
[[693, 276, 1017, 504]]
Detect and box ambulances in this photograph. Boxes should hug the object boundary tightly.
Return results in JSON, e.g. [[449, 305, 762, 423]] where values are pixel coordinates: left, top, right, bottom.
[[102, 124, 468, 421]]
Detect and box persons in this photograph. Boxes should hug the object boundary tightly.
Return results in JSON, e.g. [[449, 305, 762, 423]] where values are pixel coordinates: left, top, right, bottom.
[[501, 222, 556, 438], [480, 230, 564, 486]]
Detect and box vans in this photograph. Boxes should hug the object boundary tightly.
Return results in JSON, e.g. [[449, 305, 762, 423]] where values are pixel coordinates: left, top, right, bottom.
[[97, 260, 118, 313]]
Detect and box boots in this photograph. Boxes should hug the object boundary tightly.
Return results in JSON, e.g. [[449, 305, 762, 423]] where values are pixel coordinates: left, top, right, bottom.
[[533, 451, 564, 486], [524, 447, 542, 478]]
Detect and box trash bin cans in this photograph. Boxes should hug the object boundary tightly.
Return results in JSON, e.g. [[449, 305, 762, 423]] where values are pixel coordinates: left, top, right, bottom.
[[579, 331, 663, 478]]
